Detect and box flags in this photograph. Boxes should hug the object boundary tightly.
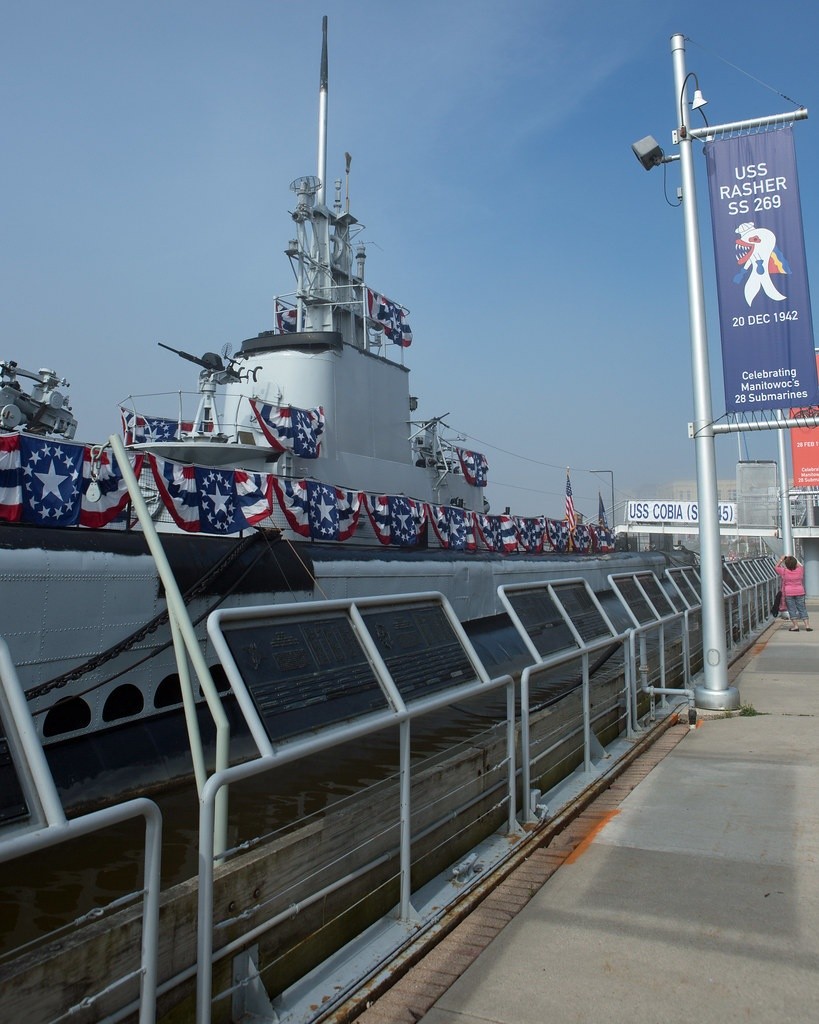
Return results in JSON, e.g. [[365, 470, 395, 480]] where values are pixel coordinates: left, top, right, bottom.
[[565, 472, 576, 531], [599, 494, 616, 547]]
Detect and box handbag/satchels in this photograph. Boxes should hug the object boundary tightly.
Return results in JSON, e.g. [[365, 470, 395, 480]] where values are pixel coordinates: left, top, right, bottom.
[[779, 593, 788, 611]]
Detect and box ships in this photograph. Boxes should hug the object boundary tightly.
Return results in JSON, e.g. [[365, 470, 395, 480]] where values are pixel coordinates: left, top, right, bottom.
[[0, 7, 784, 1024]]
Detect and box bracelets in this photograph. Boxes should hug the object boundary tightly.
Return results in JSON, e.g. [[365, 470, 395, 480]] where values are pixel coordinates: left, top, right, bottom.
[[780, 559, 782, 562]]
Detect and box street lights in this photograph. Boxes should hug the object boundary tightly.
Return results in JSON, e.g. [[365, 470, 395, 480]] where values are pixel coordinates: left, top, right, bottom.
[[589, 469, 615, 530], [632, 32, 741, 714]]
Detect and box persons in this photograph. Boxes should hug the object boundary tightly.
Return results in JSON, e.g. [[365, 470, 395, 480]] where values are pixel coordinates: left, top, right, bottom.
[[775, 555, 813, 631]]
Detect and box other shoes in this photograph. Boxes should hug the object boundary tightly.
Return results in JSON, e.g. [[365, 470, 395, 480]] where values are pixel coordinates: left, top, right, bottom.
[[806, 627, 813, 631], [789, 627, 799, 631]]
